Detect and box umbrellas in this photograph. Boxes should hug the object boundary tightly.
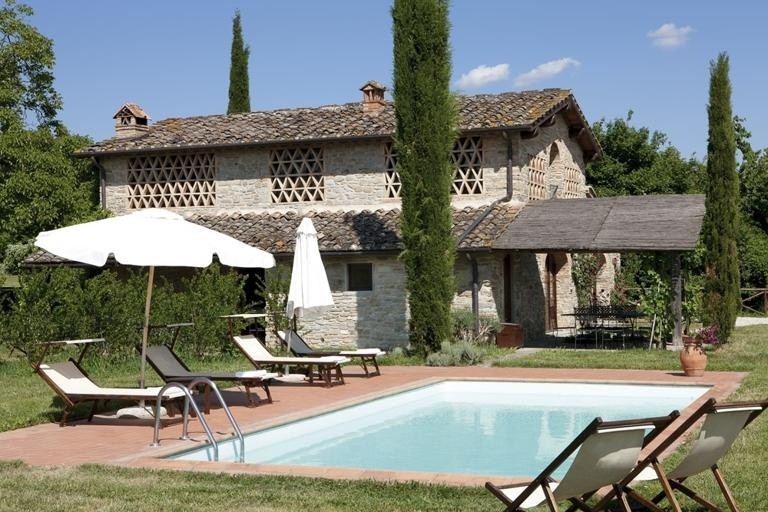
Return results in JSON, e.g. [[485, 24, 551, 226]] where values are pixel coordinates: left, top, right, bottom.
[[34, 205, 277, 408], [284, 217, 335, 377]]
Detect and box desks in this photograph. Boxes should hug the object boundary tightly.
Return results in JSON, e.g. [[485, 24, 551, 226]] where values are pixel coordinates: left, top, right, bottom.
[[560, 311, 649, 351]]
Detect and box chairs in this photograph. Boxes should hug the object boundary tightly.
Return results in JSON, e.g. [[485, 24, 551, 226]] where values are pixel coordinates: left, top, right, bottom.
[[233, 336, 351, 387], [482, 393, 766, 512], [35, 358, 198, 426], [138, 345, 279, 407], [272, 329, 386, 378], [574, 304, 637, 350]]
